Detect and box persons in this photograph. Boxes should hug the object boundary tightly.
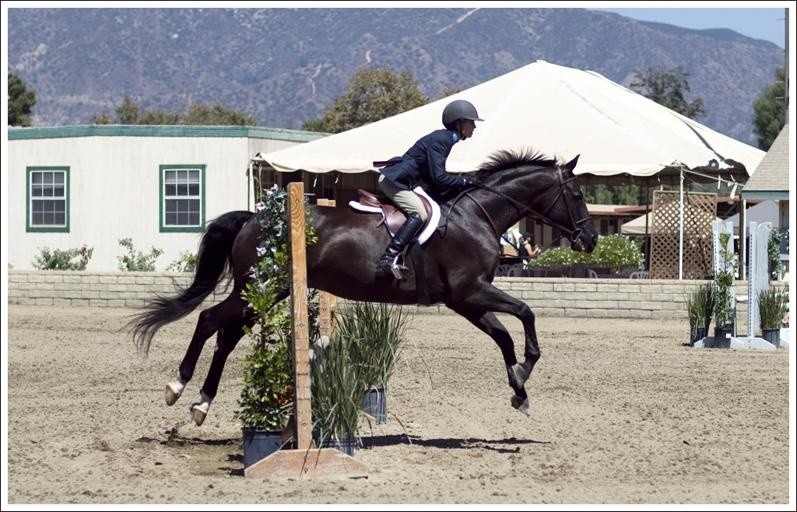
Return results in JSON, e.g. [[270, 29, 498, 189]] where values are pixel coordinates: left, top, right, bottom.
[[519, 232, 540, 258], [369, 98, 484, 279]]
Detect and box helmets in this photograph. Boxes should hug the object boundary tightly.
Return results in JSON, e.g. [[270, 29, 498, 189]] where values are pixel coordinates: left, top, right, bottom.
[[442, 101, 484, 130]]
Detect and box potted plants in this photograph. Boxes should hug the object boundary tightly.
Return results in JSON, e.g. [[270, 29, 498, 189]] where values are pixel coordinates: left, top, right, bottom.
[[231, 276, 410, 483], [683, 226, 788, 348]]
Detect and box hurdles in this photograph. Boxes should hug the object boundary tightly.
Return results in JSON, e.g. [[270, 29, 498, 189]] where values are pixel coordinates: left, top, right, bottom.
[[243, 182, 374, 482]]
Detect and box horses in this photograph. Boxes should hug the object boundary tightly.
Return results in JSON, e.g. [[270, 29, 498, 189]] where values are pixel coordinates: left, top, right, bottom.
[[116, 148, 599, 428]]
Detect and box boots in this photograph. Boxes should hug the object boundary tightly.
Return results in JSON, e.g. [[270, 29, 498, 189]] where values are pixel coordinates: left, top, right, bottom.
[[378, 212, 424, 274]]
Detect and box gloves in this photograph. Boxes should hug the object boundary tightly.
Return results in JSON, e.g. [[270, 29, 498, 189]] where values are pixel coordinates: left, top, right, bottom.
[[467, 173, 485, 188]]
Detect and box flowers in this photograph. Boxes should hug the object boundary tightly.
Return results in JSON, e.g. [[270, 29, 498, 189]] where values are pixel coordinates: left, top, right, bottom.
[[248, 183, 287, 275]]
